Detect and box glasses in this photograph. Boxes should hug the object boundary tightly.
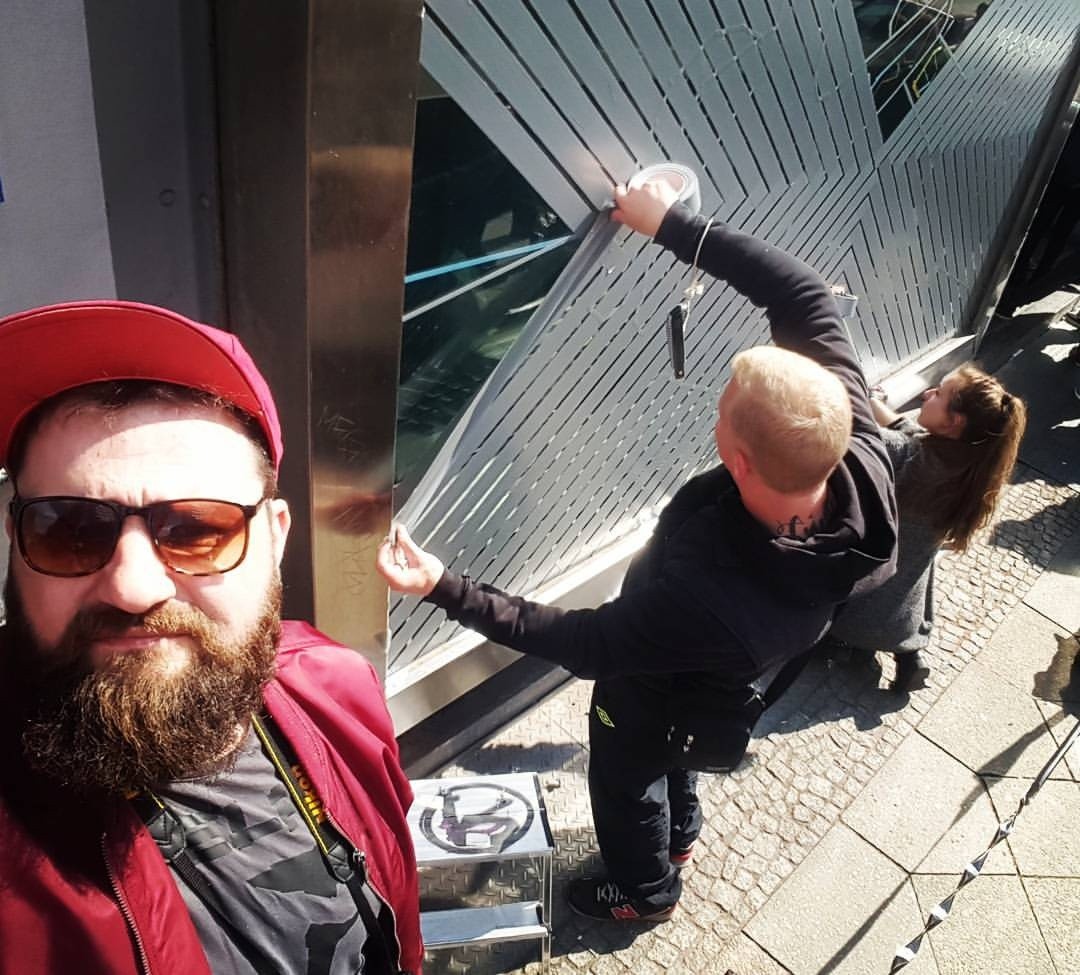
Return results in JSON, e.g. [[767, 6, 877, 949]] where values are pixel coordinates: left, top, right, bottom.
[[9, 485, 269, 578]]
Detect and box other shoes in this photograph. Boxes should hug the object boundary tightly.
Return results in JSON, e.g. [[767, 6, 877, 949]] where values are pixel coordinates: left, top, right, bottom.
[[893, 649, 930, 691]]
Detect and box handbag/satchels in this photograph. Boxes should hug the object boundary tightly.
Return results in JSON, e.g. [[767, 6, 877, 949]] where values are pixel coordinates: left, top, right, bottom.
[[651, 668, 758, 773]]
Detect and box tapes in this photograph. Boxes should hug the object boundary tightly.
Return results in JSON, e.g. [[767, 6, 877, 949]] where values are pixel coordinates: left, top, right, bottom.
[[835, 294, 858, 313], [629, 163, 703, 217]]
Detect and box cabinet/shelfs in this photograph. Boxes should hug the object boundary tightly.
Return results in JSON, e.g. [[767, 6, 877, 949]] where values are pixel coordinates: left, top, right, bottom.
[[406, 771, 554, 975]]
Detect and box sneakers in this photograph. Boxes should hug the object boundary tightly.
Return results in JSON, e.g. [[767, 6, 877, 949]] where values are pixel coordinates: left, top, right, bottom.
[[669, 842, 694, 866], [566, 873, 674, 921]]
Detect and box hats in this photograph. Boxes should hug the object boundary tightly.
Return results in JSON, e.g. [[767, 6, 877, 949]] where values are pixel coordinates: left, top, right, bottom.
[[0, 301, 284, 482]]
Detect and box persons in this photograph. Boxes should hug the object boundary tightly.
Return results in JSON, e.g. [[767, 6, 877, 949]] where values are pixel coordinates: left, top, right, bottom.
[[0, 301, 426, 975], [376, 179, 900, 922], [819, 364, 1026, 688]]
